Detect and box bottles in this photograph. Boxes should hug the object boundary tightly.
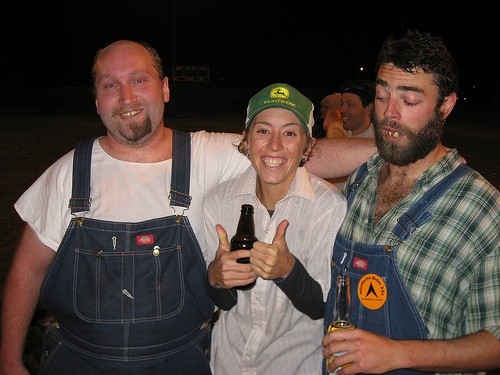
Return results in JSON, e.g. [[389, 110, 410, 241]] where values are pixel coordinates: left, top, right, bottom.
[[326, 275, 356, 373], [230, 203, 259, 290]]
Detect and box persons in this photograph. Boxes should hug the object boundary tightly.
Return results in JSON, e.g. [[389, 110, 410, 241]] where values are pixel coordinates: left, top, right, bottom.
[[321, 25, 500, 375], [321, 77, 376, 193], [201, 83, 347, 375], [0, 41, 466, 375]]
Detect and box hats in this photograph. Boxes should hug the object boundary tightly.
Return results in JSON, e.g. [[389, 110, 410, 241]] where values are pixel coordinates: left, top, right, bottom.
[[245, 83, 314, 139], [340, 80, 375, 100], [320, 92, 342, 111]]
